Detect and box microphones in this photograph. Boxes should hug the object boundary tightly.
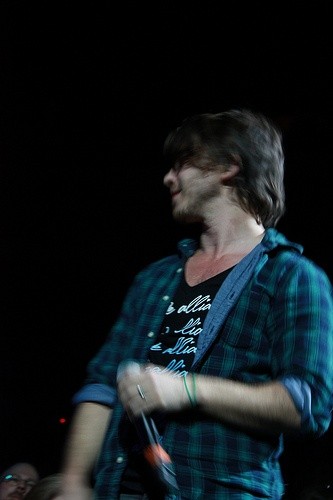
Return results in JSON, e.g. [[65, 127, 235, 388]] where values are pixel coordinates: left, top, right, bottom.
[[117, 360, 177, 489]]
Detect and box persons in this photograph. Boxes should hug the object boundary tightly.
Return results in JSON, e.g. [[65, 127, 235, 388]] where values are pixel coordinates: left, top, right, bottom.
[[53, 106, 333, 499], [0, 462, 39, 500]]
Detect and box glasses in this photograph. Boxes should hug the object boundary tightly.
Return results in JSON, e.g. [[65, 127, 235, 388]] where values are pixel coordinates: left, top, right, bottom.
[[170, 159, 219, 173], [0, 474, 37, 488]]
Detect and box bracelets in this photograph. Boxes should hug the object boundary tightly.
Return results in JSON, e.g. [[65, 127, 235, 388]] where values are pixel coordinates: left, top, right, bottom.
[[191, 373, 199, 406], [184, 374, 193, 408]]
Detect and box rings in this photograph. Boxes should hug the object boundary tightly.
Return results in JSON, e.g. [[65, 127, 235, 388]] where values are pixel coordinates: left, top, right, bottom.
[[138, 387, 147, 400]]
